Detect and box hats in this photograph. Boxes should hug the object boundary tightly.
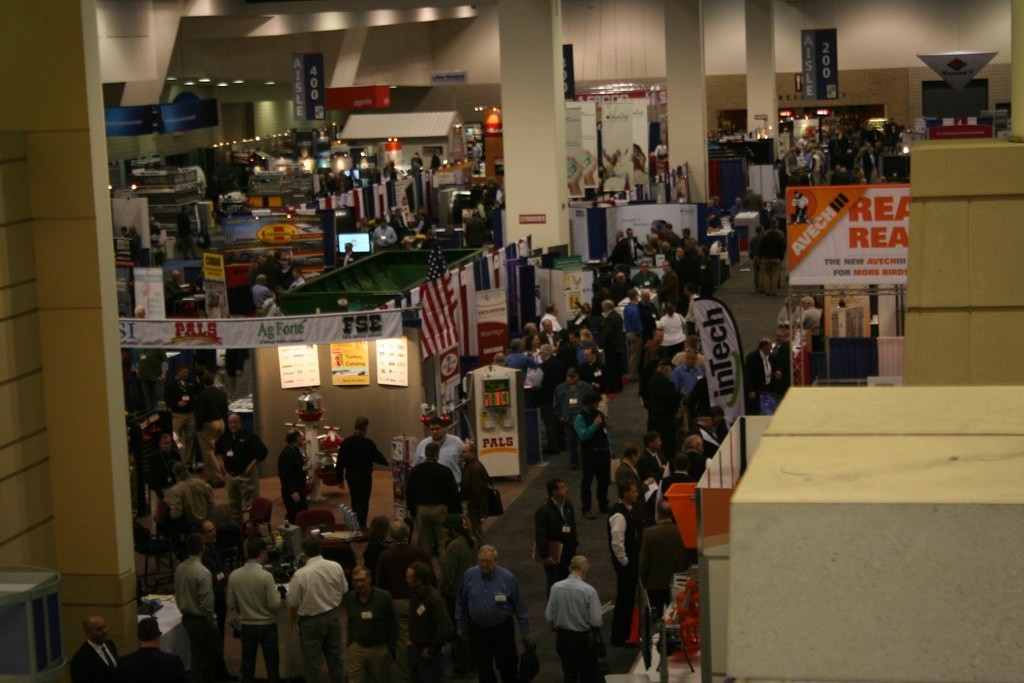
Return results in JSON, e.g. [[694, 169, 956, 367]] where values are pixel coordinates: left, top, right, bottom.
[[580, 391, 602, 403], [580, 329, 592, 341], [137, 617, 159, 632], [441, 513, 464, 529], [700, 408, 714, 417], [566, 367, 578, 376]]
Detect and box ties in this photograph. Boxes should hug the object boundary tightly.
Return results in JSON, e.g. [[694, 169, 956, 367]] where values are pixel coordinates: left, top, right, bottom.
[[101, 646, 114, 672]]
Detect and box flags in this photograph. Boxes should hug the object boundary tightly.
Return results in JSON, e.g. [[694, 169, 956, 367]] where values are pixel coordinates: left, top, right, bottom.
[[420, 237, 460, 360]]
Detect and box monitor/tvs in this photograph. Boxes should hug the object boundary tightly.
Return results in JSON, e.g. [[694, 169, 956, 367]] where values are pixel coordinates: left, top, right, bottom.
[[106, 98, 219, 136], [882, 155, 910, 179], [339, 233, 370, 253], [344, 169, 360, 180]]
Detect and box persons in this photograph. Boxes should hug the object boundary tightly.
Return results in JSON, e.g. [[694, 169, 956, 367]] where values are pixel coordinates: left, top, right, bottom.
[[177, 205, 202, 260], [77, 132, 884, 682]]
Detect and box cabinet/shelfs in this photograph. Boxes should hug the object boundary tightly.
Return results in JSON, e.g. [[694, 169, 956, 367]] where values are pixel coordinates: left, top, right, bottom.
[[127, 168, 204, 234]]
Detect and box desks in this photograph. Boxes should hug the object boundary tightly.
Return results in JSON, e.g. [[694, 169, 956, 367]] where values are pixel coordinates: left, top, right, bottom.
[[172, 294, 206, 318], [137, 594, 192, 672], [630, 261, 667, 280], [277, 523, 305, 564], [431, 227, 466, 249], [707, 229, 739, 287], [733, 211, 760, 243], [317, 524, 368, 574], [228, 397, 255, 434]]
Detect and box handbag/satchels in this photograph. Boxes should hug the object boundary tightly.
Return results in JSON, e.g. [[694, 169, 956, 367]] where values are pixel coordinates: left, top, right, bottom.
[[516, 641, 541, 683], [592, 627, 607, 658], [592, 345, 607, 366], [481, 483, 504, 516]]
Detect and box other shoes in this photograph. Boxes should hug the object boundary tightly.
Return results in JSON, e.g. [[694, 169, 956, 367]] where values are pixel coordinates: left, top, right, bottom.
[[610, 639, 627, 647], [583, 511, 597, 519], [208, 480, 226, 488]]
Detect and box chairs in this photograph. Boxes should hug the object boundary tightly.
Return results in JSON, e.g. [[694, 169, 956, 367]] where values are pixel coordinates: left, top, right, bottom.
[[215, 496, 275, 572], [295, 509, 336, 530], [131, 523, 180, 589]]
